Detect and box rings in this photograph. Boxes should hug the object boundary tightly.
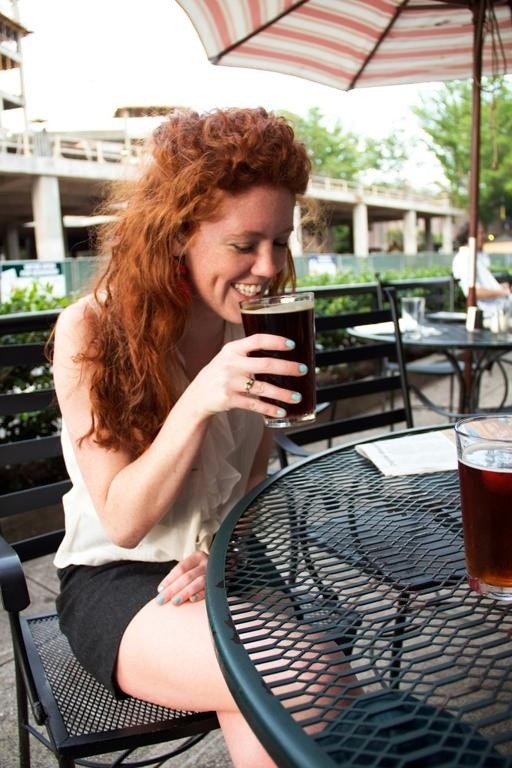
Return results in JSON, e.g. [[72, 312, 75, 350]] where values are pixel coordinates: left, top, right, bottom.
[[243, 376, 255, 394]]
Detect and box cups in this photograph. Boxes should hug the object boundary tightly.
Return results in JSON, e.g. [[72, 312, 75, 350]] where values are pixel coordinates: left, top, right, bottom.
[[401, 297, 426, 339], [496, 297, 512, 344], [238, 292, 318, 431], [452, 411, 511, 600]]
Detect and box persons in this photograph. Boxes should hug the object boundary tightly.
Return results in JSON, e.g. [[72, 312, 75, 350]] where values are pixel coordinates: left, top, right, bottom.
[[453, 221, 512, 330], [53, 108, 365, 768]]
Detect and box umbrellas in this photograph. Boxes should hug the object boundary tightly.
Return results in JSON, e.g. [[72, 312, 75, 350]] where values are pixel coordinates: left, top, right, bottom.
[[175, 1, 512, 386]]
